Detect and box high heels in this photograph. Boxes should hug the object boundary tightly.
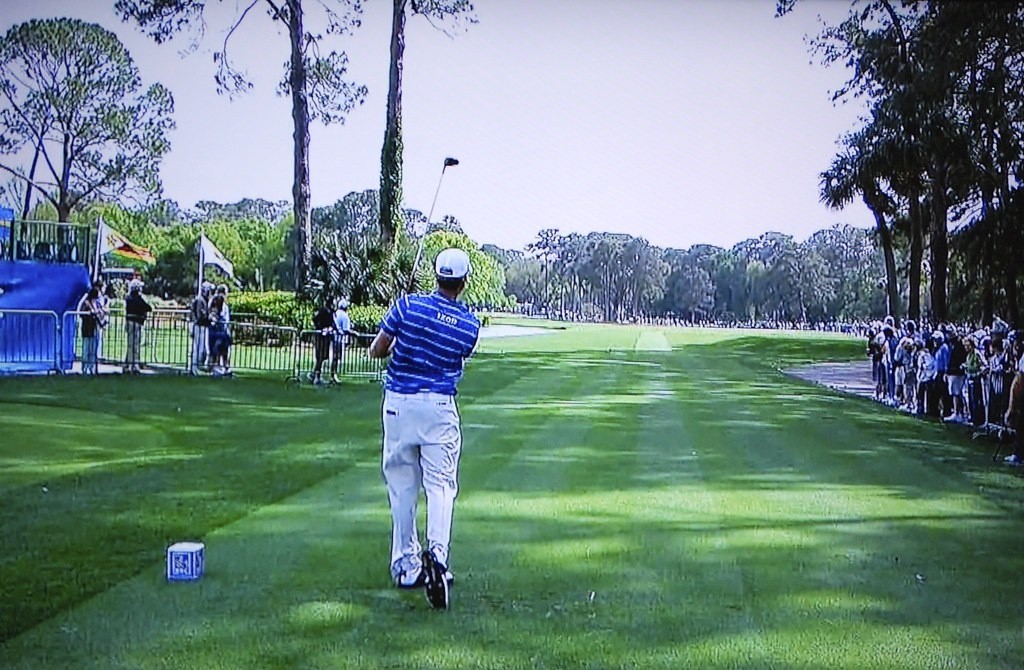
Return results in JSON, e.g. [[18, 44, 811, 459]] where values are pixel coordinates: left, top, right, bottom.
[[330, 373, 342, 385]]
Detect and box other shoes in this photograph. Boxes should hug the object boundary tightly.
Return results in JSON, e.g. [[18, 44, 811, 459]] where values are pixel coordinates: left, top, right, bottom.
[[312, 379, 327, 385], [944, 414, 962, 422]]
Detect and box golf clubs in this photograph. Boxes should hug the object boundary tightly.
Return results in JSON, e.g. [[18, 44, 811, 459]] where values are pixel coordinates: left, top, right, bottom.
[[405, 155, 461, 294], [992, 412, 1018, 463]]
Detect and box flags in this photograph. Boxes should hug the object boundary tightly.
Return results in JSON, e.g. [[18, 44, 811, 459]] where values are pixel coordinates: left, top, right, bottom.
[[100, 221, 156, 266], [202, 234, 234, 279]]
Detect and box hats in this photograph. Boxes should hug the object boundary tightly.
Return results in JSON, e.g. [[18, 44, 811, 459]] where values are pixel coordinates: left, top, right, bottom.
[[202, 281, 216, 292], [931, 330, 945, 342], [130, 279, 145, 289], [434, 247, 470, 280], [338, 299, 350, 309]]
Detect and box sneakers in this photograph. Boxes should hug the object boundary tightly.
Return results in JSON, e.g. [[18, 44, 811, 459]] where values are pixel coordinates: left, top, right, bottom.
[[395, 565, 454, 589], [421, 545, 449, 610]]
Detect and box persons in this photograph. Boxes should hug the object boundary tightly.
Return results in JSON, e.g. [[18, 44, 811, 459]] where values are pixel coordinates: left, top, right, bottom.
[[634, 315, 867, 337], [79, 280, 110, 375], [188, 281, 233, 380], [370, 247, 482, 611], [307, 295, 359, 386], [867, 315, 1024, 464], [124, 279, 152, 373]]
